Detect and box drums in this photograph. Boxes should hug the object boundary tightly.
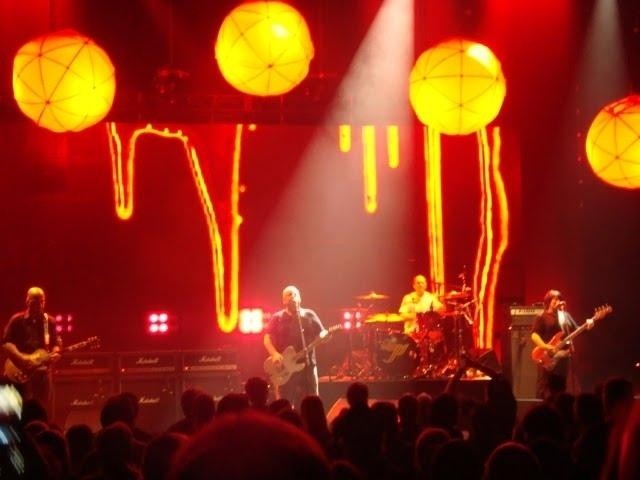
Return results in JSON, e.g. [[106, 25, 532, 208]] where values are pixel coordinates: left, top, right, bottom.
[[377, 332, 430, 379], [416, 311, 442, 331], [365, 320, 405, 365]]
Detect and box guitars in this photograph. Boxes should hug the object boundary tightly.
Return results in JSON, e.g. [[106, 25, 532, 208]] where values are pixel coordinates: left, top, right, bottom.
[[531, 303, 613, 372], [263, 323, 342, 385], [5, 336, 101, 383]]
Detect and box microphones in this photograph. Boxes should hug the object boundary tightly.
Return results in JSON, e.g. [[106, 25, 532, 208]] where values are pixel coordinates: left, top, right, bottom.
[[559, 301, 566, 305], [290, 298, 297, 307]]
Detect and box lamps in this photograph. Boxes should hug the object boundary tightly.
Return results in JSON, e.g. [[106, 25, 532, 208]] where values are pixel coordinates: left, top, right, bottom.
[[581, 91, 640, 190], [213, 0, 315, 96], [12, 1, 118, 133], [408, 5, 508, 138]]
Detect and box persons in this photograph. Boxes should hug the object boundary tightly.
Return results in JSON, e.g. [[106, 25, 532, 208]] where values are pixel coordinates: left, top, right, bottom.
[[3, 286, 70, 426], [531, 290, 594, 397], [0, 372, 640, 480], [264, 284, 332, 409], [399, 274, 447, 335]]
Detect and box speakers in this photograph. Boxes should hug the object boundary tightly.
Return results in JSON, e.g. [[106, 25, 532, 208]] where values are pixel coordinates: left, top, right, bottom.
[[54, 347, 241, 424]]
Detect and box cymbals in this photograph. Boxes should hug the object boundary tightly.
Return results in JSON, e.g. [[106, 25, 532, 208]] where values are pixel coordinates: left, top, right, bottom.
[[438, 290, 466, 301], [352, 293, 389, 300], [442, 310, 466, 316]]
